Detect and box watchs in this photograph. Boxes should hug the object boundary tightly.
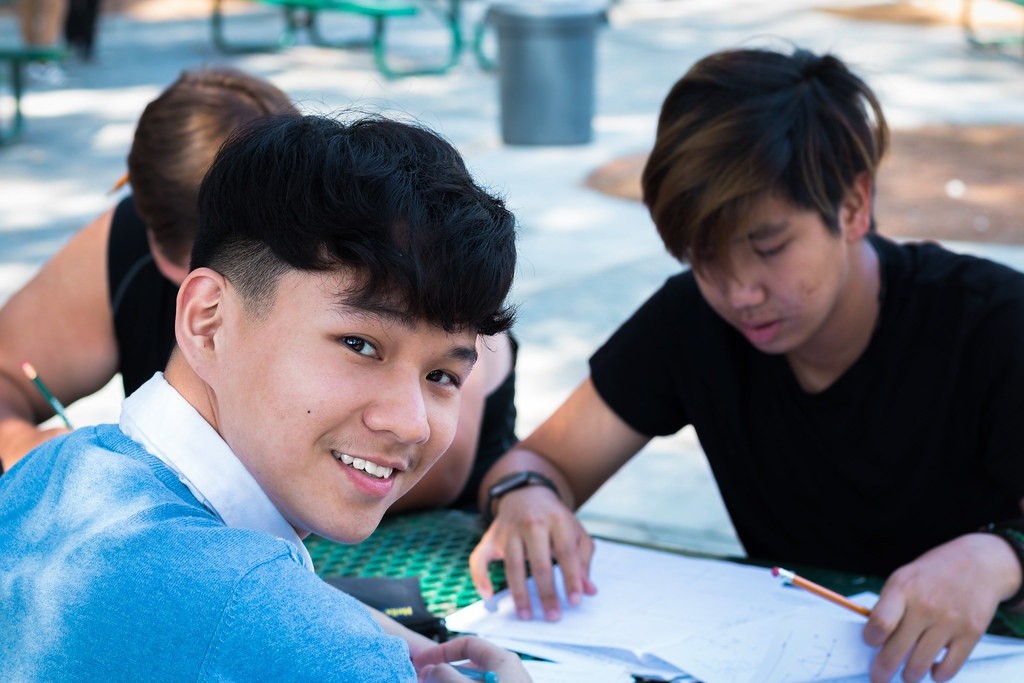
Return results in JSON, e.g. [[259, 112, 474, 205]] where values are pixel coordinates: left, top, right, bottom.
[[484, 471, 568, 520]]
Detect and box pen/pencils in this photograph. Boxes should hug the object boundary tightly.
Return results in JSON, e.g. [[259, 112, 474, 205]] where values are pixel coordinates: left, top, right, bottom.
[[772, 566, 873, 618], [20, 361, 78, 431]]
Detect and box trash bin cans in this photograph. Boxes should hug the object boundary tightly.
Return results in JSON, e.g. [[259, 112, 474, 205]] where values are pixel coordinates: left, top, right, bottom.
[[490, 2, 610, 146]]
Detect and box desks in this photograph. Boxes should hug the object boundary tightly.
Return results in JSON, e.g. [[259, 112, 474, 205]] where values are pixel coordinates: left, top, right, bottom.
[[300, 512, 1023, 683]]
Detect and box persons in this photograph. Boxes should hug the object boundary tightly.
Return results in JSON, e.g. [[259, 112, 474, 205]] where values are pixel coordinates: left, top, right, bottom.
[[2, 65, 517, 522], [466, 45, 1024, 683], [0, 112, 534, 679]]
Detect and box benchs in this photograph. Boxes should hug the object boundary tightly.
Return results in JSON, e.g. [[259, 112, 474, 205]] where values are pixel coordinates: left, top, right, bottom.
[[210, 0, 465, 78], [0, 46, 62, 149]]
